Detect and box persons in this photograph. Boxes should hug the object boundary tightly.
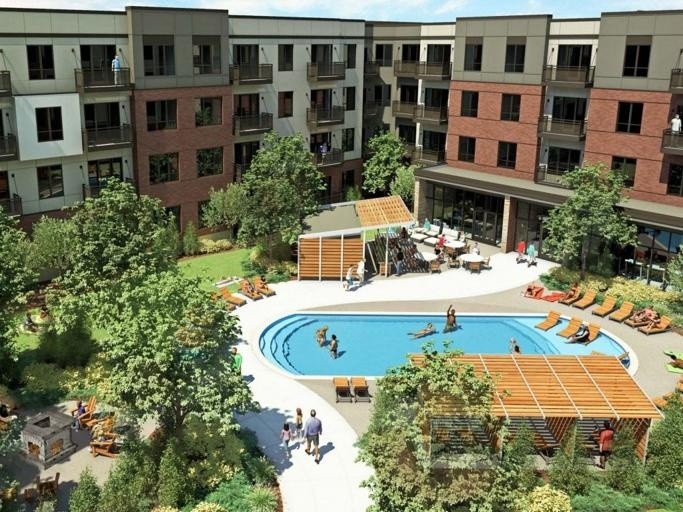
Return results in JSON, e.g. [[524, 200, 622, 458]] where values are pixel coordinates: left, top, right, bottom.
[[258, 275, 268, 290], [342, 264, 354, 290], [444, 304, 457, 332], [110, 55, 121, 85], [70, 399, 85, 429], [523, 285, 543, 297], [376, 217, 479, 269], [628, 303, 654, 323], [395, 248, 403, 276], [563, 321, 590, 343], [320, 143, 327, 165], [517, 238, 525, 263], [302, 409, 321, 464], [356, 258, 367, 286], [279, 421, 293, 458], [647, 313, 661, 330], [668, 354, 682, 367], [598, 421, 614, 468], [670, 113, 682, 148], [526, 241, 536, 265], [414, 322, 433, 337], [558, 281, 578, 303], [510, 337, 520, 355], [294, 407, 304, 439], [328, 334, 336, 359], [315, 325, 328, 345]]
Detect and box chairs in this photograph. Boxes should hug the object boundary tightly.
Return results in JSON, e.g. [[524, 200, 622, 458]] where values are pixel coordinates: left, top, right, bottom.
[[536, 284, 683, 411], [0, 397, 120, 502], [411, 224, 491, 274], [351, 376, 370, 403], [333, 376, 352, 404], [435, 427, 602, 451], [209, 276, 277, 311]]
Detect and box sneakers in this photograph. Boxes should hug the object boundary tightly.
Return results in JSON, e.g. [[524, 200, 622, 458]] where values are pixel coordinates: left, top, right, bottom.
[[305, 449, 311, 455], [315, 459, 319, 464]]
